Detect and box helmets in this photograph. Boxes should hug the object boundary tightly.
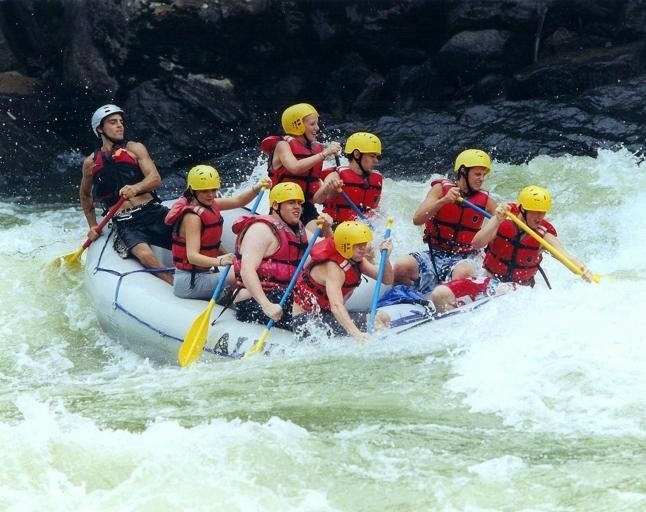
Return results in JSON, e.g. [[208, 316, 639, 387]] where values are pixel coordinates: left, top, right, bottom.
[[187, 164, 221, 192], [281, 103, 318, 137], [454, 149, 491, 174], [92, 104, 124, 139], [334, 221, 372, 259], [517, 186, 551, 211], [270, 182, 305, 208], [344, 131, 381, 155]]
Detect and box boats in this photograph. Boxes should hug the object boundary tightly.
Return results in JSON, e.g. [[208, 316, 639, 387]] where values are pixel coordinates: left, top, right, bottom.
[[86, 194, 526, 371]]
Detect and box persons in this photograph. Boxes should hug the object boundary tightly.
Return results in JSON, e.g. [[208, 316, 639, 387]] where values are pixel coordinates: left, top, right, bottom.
[[79, 104, 227, 286], [292, 221, 394, 344], [432, 185, 593, 314], [261, 102, 340, 234], [313, 132, 384, 233], [164, 164, 273, 299], [393, 148, 499, 294], [232, 181, 334, 332]]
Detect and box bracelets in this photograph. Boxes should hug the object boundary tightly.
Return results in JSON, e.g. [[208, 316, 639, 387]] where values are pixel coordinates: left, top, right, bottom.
[[252, 186, 258, 194], [319, 152, 327, 159], [219, 258, 223, 265]]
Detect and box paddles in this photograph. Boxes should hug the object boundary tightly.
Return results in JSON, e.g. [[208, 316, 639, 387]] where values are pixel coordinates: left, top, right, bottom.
[[243, 220, 323, 360], [43, 194, 127, 275], [178, 185, 268, 368]]
